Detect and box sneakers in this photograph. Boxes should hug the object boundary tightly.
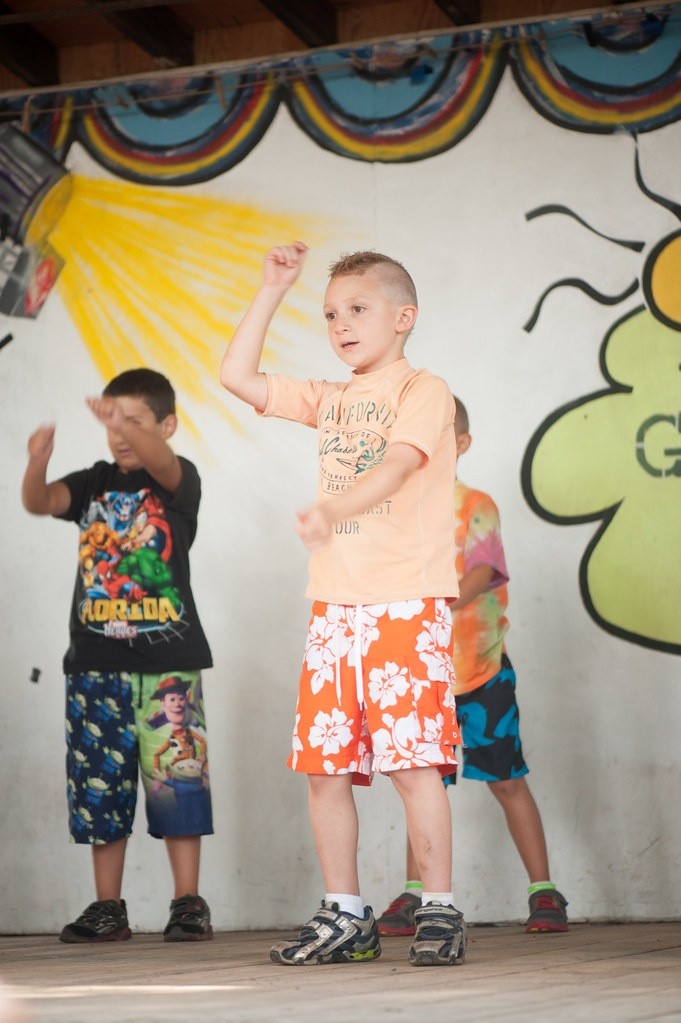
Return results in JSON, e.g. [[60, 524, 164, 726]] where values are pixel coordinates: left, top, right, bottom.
[[270, 899, 382, 965], [409, 900, 468, 965], [374, 892, 421, 935], [59, 899, 132, 942], [526, 889, 568, 932], [163, 894, 213, 942]]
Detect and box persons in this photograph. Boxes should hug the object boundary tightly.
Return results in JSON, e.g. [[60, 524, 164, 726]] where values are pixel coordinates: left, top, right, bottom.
[[220, 240, 467, 966], [378, 396, 571, 935], [21, 369, 216, 942]]
